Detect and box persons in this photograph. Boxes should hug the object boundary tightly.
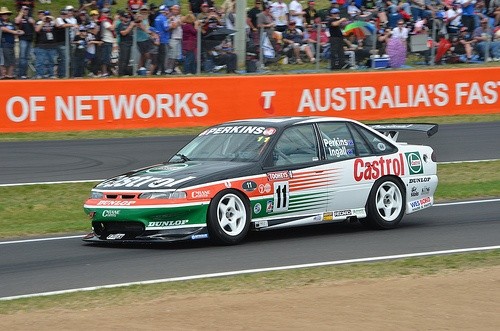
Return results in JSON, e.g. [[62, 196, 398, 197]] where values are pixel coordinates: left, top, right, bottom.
[[0, 0, 500, 79]]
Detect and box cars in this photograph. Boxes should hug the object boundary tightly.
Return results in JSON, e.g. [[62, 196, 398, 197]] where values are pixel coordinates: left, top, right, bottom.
[[81, 117, 439, 246]]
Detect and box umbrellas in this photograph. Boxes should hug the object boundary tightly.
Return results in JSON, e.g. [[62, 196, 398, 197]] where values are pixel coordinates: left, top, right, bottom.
[[344, 21, 375, 47]]
